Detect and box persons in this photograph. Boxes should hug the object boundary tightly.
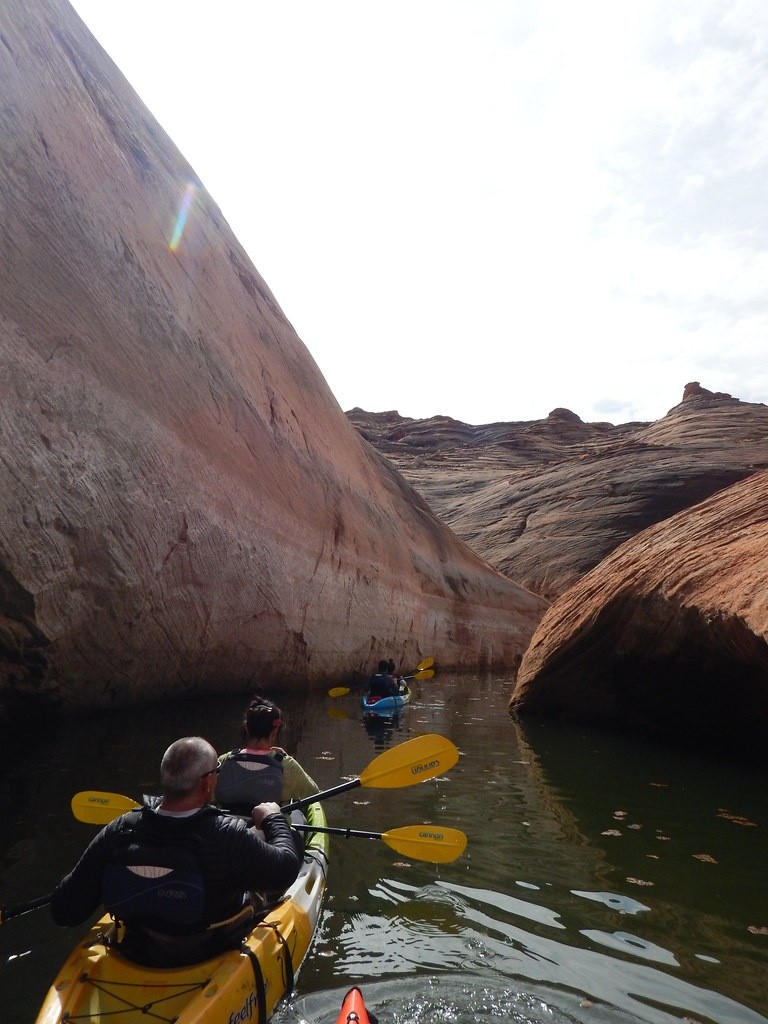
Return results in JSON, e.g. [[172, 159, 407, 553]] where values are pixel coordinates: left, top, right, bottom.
[[50, 737, 303, 972], [368, 658, 408, 699], [205, 696, 308, 849]]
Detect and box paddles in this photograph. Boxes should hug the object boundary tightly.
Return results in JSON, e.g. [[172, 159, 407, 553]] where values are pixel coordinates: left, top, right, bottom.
[[400, 656, 434, 678], [0, 733, 460, 924], [70, 789, 467, 863], [329, 670, 435, 698]]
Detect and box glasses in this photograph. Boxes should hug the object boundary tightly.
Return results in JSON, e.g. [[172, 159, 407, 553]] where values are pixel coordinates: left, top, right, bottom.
[[201, 761, 222, 779]]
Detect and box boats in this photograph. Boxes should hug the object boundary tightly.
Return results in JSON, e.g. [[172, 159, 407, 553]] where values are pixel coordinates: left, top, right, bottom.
[[360, 682, 413, 710], [30, 748, 330, 1024]]
